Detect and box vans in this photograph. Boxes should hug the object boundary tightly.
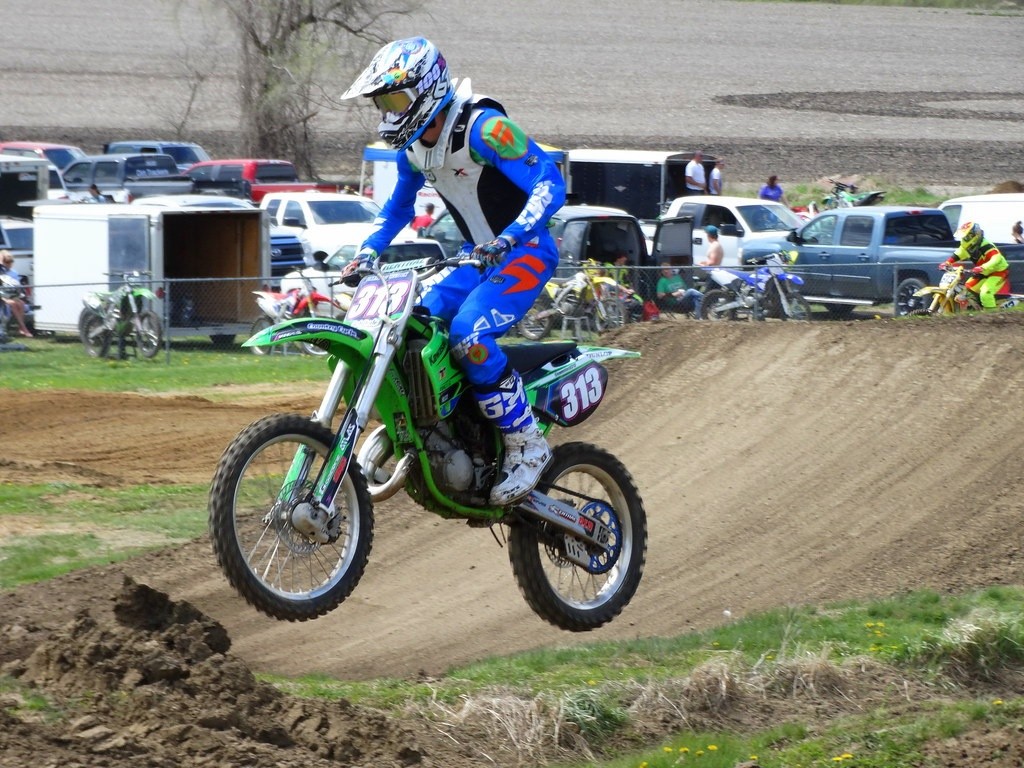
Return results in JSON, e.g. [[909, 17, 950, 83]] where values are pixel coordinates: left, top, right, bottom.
[[549, 205, 649, 293], [925, 193, 1024, 245]]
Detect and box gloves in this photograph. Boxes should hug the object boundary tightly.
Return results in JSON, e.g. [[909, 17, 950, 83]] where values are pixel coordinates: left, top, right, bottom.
[[342, 249, 378, 287], [972, 267, 982, 276], [939, 261, 948, 270], [471, 236, 511, 275]]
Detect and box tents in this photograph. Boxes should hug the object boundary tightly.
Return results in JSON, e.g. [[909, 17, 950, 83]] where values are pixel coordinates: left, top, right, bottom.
[[359, 141, 570, 203]]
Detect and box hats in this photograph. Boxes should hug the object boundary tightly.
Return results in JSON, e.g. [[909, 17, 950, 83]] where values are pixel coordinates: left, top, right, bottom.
[[705, 225, 718, 235]]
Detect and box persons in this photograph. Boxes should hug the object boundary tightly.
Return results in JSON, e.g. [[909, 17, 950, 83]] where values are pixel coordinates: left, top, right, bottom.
[[656, 262, 705, 320], [684, 150, 708, 195], [759, 175, 791, 208], [339, 37, 567, 504], [87, 183, 105, 203], [605, 255, 630, 288], [709, 158, 725, 196], [0, 249, 34, 337], [412, 203, 436, 231], [940, 221, 1009, 308], [699, 225, 723, 291]]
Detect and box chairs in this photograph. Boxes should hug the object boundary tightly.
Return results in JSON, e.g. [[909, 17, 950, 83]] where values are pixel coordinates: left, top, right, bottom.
[[642, 270, 697, 321]]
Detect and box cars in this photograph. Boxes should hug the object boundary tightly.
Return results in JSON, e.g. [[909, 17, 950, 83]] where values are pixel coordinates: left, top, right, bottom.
[[0, 140, 468, 299]]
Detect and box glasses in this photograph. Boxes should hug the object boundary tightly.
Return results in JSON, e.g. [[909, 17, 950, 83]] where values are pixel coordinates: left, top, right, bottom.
[[5, 260, 14, 264], [374, 88, 418, 115]]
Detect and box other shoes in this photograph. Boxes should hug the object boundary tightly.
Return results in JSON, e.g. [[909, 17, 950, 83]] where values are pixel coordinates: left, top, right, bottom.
[[19, 328, 32, 337]]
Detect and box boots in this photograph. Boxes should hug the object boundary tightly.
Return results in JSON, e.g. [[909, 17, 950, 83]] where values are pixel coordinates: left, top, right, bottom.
[[472, 361, 557, 505]]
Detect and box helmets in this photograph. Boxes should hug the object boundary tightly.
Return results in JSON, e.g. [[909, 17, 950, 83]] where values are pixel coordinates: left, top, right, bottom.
[[953, 222, 984, 251], [340, 36, 456, 151]]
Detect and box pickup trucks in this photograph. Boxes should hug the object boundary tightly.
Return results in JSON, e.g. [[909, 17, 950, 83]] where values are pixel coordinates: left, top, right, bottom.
[[636, 196, 818, 271], [737, 204, 1024, 318]]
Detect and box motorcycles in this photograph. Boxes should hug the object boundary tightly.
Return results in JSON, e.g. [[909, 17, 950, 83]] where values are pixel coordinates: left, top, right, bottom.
[[208, 249, 648, 633], [515, 256, 628, 342], [700, 248, 813, 322], [904, 264, 1024, 319], [821, 178, 888, 207], [78, 270, 165, 359]]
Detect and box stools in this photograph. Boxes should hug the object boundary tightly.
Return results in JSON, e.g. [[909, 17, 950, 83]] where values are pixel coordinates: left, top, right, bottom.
[[561, 316, 592, 344]]
